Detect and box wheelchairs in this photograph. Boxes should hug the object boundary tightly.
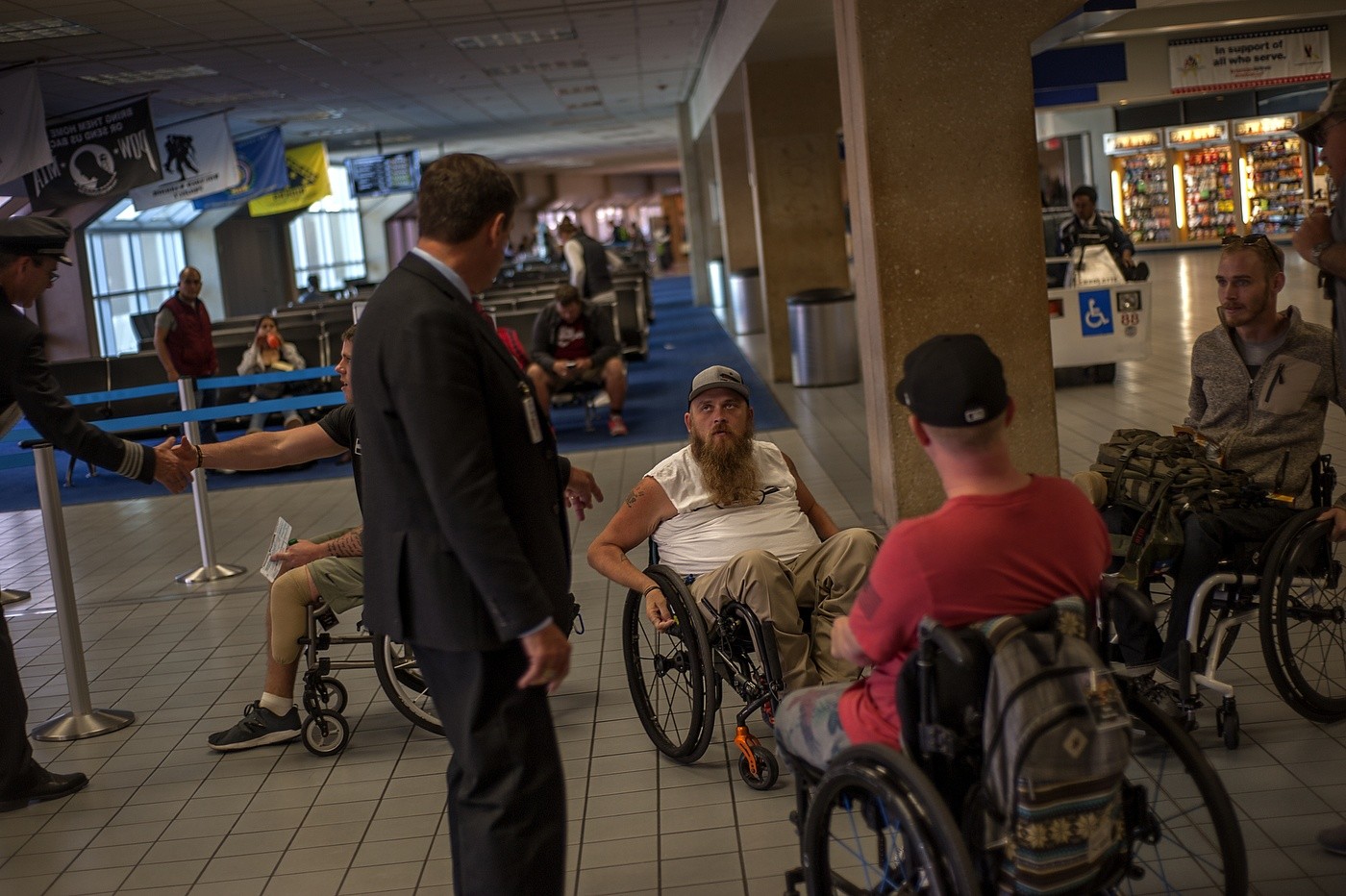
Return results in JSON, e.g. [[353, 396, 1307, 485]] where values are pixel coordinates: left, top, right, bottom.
[[780, 572, 1250, 896], [1077, 426, 1345, 745], [297, 598, 447, 757], [623, 530, 786, 791]]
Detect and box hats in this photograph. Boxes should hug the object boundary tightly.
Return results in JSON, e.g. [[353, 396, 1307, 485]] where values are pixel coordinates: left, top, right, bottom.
[[688, 365, 750, 406], [894, 332, 1007, 428], [1, 215, 75, 266], [1291, 79, 1346, 147]]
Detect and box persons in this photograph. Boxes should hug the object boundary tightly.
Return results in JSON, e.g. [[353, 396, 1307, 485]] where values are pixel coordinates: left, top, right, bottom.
[[1058, 183, 1150, 281], [1290, 75, 1346, 421], [496, 205, 676, 345], [235, 312, 307, 435], [350, 148, 609, 894], [525, 285, 633, 436], [170, 321, 366, 751], [154, 264, 239, 479], [769, 332, 1115, 801], [587, 364, 886, 702], [1090, 232, 1346, 756], [0, 216, 193, 810]]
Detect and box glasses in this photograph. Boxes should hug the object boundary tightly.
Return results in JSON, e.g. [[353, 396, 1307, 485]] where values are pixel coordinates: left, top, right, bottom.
[[1314, 117, 1345, 145], [33, 263, 59, 283], [1220, 233, 1282, 271]]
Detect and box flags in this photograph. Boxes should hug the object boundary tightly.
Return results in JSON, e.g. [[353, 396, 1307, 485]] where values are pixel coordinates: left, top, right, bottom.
[[1, 74, 431, 230]]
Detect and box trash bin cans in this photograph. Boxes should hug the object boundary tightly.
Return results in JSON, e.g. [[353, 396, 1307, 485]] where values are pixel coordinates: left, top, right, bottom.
[[788, 287, 862, 388], [730, 267, 766, 335], [709, 258, 728, 307]]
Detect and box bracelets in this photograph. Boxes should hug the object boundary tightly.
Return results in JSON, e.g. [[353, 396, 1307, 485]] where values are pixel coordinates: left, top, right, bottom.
[[643, 585, 660, 598], [192, 441, 204, 469]]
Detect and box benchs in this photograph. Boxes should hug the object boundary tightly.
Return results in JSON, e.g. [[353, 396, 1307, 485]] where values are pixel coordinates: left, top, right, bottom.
[[21, 258, 671, 461]]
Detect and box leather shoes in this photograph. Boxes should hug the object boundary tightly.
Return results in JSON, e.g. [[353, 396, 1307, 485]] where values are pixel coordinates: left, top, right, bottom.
[[0, 761, 91, 814]]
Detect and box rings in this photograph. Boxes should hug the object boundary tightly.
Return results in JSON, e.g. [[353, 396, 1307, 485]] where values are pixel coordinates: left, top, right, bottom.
[[571, 495, 577, 500]]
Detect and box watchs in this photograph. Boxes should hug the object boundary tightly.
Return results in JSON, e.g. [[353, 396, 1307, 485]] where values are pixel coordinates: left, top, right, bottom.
[[1310, 238, 1336, 266]]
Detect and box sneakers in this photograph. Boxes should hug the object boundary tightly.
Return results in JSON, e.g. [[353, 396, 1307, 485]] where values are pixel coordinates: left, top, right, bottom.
[[207, 702, 304, 750], [607, 417, 628, 436]]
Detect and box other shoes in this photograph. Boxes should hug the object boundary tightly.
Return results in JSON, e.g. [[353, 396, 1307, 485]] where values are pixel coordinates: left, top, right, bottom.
[[284, 416, 303, 430], [1130, 666, 1196, 719], [1317, 824, 1346, 856]]
